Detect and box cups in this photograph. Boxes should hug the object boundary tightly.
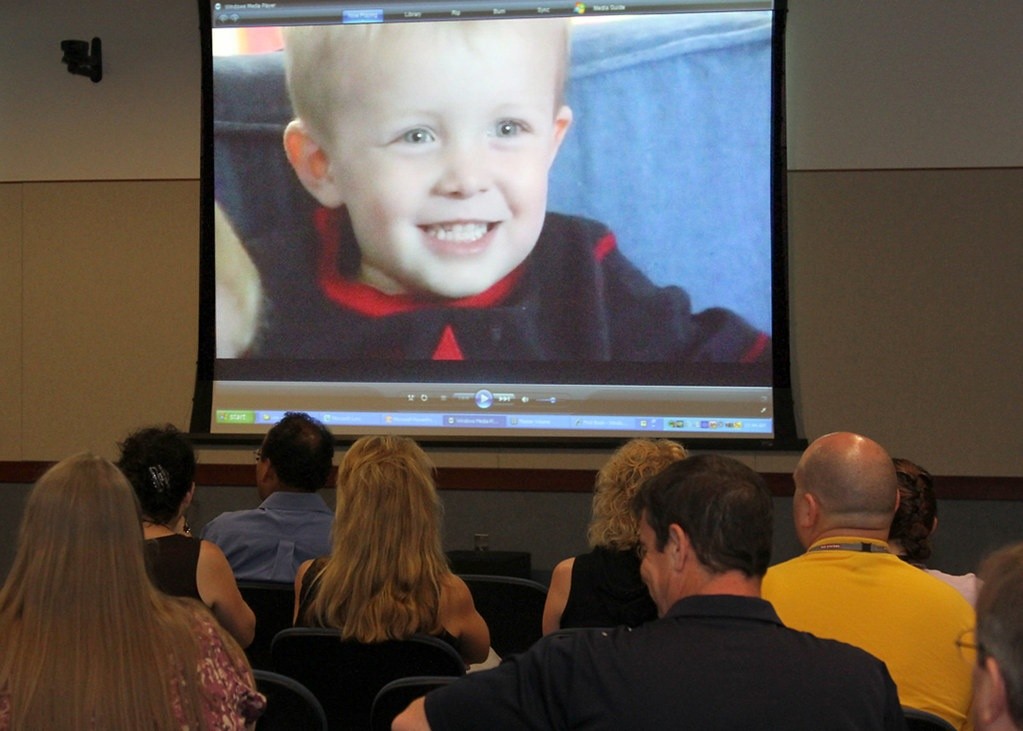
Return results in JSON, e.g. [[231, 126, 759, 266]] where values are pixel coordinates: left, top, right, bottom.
[[475, 534, 487, 552]]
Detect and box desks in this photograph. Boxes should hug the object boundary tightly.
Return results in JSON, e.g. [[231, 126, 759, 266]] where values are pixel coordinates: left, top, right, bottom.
[[444, 549, 532, 575]]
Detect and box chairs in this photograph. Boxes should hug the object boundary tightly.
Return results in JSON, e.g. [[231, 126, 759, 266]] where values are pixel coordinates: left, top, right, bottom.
[[458, 575, 548, 656], [254, 625, 468, 731], [235, 580, 299, 623]]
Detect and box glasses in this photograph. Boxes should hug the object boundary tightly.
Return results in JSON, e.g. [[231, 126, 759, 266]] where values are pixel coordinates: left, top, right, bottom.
[[253, 449, 266, 460], [955, 629, 993, 667]]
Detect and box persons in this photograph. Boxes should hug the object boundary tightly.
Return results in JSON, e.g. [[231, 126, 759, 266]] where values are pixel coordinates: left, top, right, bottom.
[[0, 451, 266, 731], [761, 432, 977, 731], [119, 424, 256, 650], [214, 20, 771, 363], [542, 439, 687, 637], [887, 457, 984, 607], [198, 412, 336, 582], [293, 435, 490, 670], [968, 545, 1023, 731], [391, 454, 908, 731]]
